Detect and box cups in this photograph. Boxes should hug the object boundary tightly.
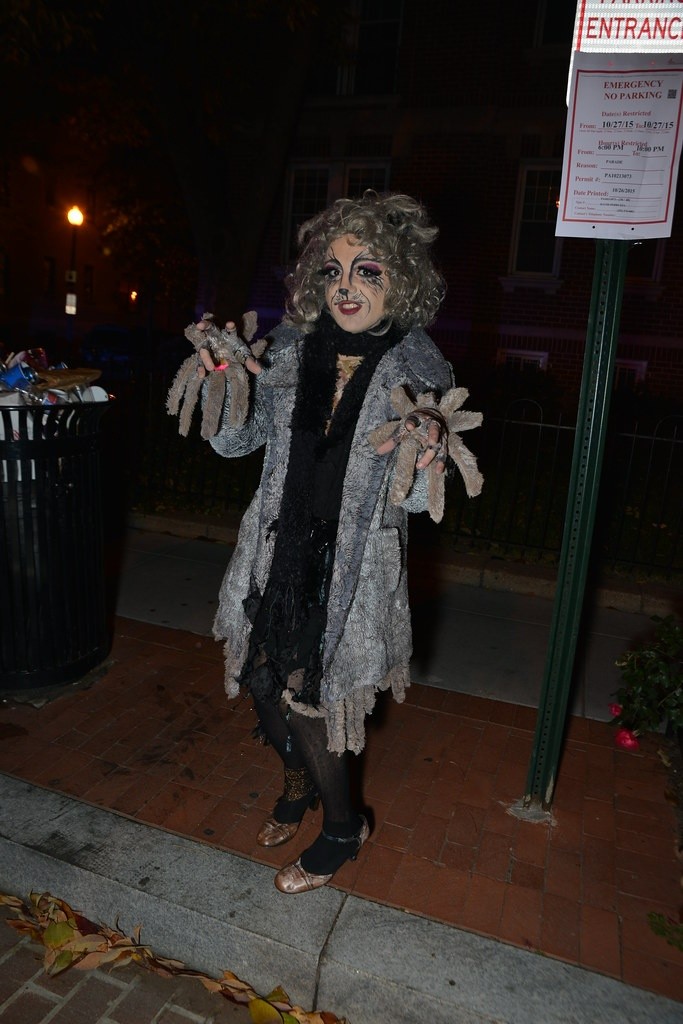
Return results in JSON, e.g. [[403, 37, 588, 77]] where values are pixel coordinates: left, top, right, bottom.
[[72, 384, 95, 403], [4, 361, 37, 391], [56, 361, 68, 369]]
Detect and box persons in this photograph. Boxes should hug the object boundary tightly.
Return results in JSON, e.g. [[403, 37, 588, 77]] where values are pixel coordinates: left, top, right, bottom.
[[167, 192, 482, 893]]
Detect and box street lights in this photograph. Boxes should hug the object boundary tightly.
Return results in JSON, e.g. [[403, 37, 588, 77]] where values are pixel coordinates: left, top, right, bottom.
[[65, 204, 86, 338]]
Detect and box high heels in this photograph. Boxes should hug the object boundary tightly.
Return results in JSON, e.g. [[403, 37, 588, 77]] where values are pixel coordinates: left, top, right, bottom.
[[257, 789, 321, 847], [274, 816, 370, 895]]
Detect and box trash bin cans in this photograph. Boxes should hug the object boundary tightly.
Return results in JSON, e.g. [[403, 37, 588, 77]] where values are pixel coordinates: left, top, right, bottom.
[[0, 393, 121, 690]]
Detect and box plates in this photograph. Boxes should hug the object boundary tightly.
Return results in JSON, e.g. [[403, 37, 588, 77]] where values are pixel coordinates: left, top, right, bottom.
[[83, 386, 108, 402]]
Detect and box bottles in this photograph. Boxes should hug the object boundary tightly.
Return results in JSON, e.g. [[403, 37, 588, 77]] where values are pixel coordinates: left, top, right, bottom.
[[16, 358, 39, 385], [13, 384, 67, 416]]
